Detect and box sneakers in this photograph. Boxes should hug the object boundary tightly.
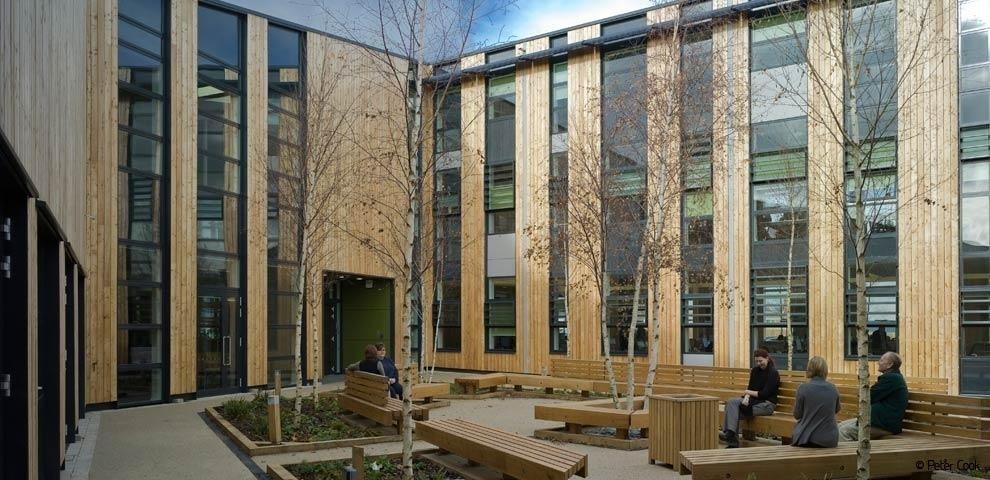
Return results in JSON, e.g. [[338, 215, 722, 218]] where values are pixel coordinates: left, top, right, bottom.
[[720, 432, 739, 448]]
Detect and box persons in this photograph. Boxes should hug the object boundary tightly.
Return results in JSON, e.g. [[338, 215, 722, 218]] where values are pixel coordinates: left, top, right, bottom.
[[374, 343, 403, 402], [719, 349, 781, 448], [871, 326, 888, 355], [344, 345, 397, 399], [790, 356, 842, 448], [837, 350, 908, 443]]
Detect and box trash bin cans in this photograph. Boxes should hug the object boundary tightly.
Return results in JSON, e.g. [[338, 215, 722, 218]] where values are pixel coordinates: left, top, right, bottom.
[[647, 393, 721, 471]]
